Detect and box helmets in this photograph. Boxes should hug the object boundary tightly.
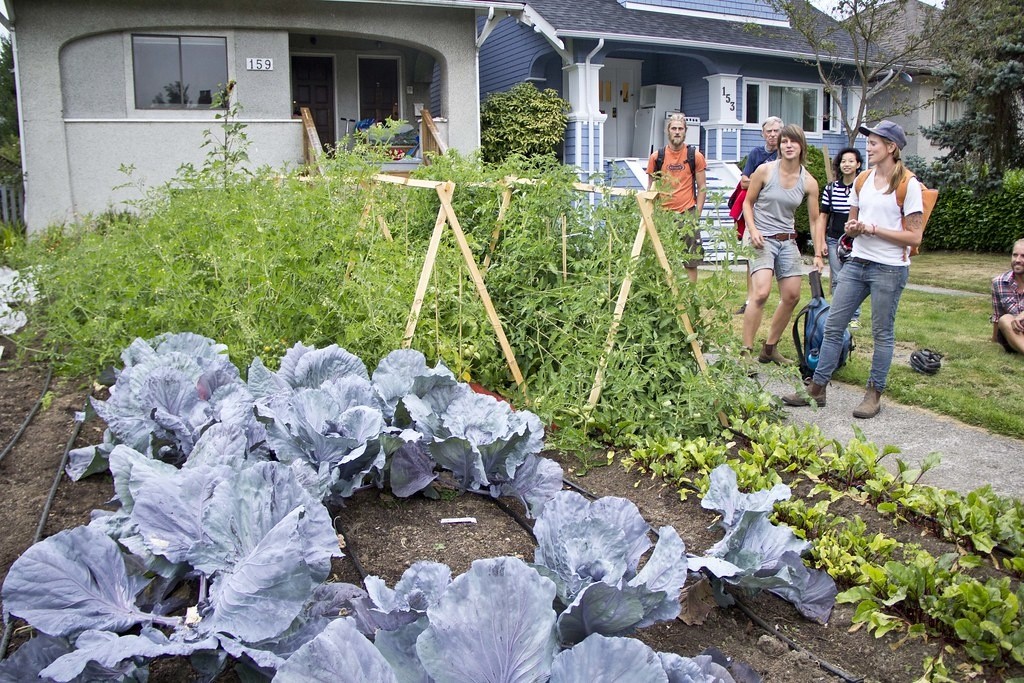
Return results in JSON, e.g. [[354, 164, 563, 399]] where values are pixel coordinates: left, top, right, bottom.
[[910, 350, 941, 376]]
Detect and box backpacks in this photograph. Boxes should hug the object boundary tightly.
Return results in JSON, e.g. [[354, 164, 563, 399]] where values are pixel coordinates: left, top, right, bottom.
[[855, 169, 938, 262], [793, 270, 857, 387]]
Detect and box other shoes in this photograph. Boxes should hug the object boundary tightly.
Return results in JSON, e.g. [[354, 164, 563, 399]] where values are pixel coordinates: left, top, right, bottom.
[[849, 321, 863, 330], [737, 304, 746, 313]]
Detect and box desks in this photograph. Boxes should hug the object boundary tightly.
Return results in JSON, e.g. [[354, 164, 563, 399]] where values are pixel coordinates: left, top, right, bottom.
[[417, 119, 448, 159]]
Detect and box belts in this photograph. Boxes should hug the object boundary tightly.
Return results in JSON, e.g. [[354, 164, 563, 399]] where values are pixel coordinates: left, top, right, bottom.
[[763, 233, 798, 241]]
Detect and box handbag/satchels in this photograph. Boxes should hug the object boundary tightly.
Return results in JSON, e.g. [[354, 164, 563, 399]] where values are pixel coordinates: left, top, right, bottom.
[[734, 217, 745, 241], [355, 118, 375, 129]]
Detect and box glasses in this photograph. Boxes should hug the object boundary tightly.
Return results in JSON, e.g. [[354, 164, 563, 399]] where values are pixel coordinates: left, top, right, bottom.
[[922, 349, 943, 362]]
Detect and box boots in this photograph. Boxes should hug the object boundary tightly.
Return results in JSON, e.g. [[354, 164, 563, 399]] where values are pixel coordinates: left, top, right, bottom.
[[740, 348, 753, 356], [853, 380, 881, 418], [782, 380, 826, 406], [758, 339, 794, 368]]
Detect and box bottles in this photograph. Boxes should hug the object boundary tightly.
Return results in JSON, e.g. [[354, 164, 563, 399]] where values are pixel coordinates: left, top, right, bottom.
[[808, 348, 819, 369]]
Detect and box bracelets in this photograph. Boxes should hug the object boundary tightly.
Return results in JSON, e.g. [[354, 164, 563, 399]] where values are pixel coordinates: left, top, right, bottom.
[[872, 224, 876, 236], [815, 255, 821, 258]]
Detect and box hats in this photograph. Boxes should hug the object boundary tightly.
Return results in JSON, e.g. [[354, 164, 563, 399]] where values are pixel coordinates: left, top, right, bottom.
[[858, 120, 907, 150]]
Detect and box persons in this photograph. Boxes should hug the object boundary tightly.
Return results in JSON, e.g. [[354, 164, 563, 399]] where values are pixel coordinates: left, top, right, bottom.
[[819, 147, 864, 328], [782, 121, 923, 419], [646, 114, 707, 281], [737, 115, 784, 313], [990, 237, 1024, 357], [744, 124, 825, 368]]
